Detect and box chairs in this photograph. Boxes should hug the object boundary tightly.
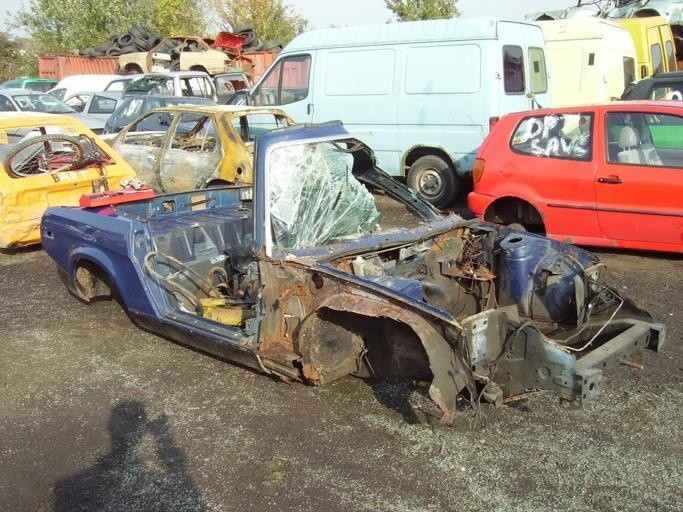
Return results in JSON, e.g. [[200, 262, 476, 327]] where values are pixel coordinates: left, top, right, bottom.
[[617, 119, 663, 165]]
